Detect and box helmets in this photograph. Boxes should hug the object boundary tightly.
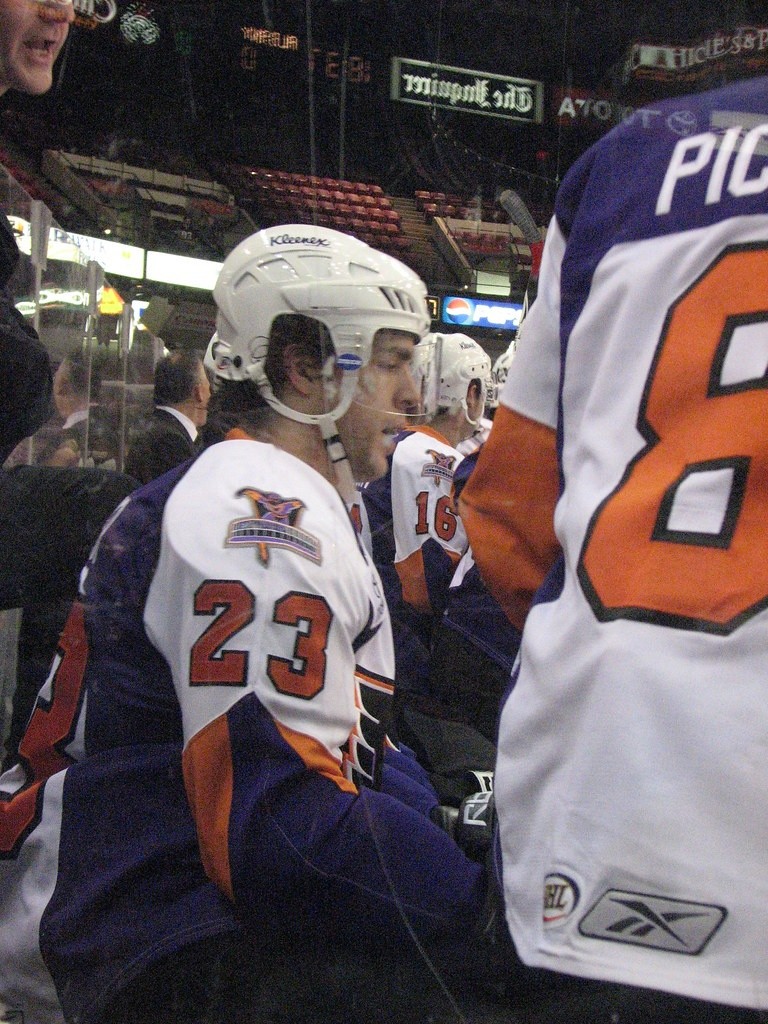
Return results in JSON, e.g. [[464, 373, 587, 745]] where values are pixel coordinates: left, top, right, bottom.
[[212, 223, 432, 426], [203, 330, 249, 381], [490, 350, 516, 408], [413, 330, 492, 408]]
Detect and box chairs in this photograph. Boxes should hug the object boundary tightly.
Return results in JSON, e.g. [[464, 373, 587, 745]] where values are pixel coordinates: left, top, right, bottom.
[[0, 147, 231, 226], [232, 160, 516, 267]]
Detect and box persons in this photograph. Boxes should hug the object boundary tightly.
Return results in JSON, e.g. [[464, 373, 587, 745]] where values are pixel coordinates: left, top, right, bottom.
[[0, 0, 768, 1024], [0, 345, 122, 775]]
